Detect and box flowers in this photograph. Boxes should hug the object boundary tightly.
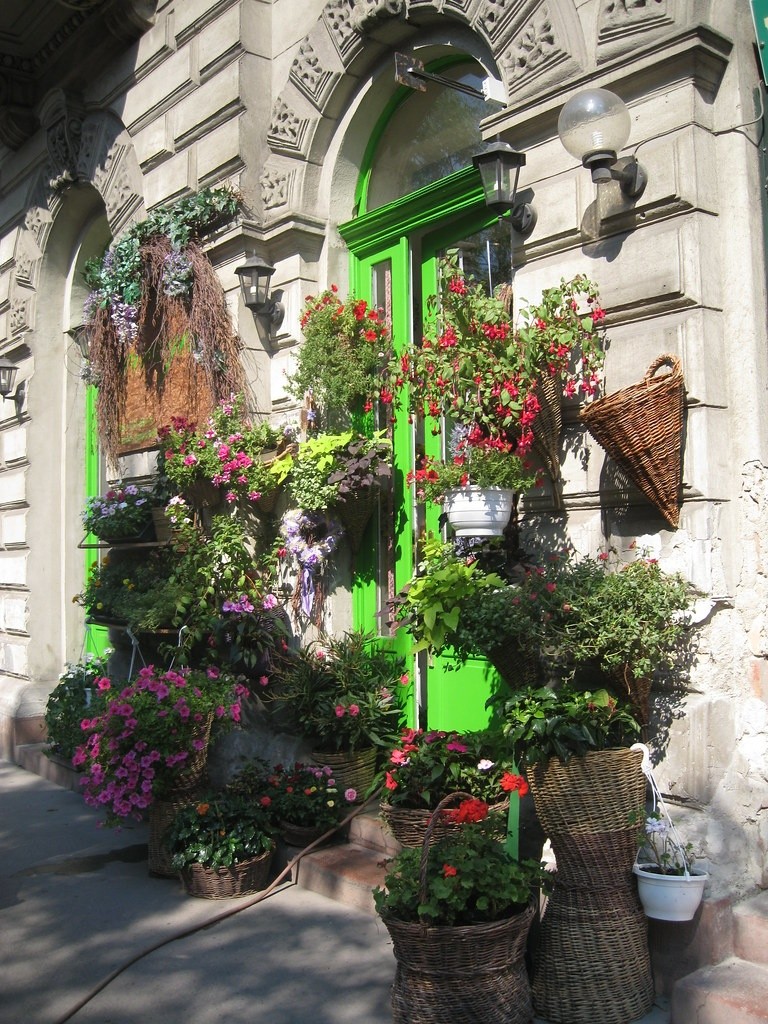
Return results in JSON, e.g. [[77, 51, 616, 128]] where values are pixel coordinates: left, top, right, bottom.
[[407, 426, 547, 504], [42, 184, 693, 926]]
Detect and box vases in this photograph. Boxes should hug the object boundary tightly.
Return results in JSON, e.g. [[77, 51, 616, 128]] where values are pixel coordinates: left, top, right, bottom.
[[444, 487, 516, 540], [85, 688, 98, 705], [152, 507, 174, 543], [634, 864, 708, 921], [85, 608, 128, 625], [104, 521, 155, 543]]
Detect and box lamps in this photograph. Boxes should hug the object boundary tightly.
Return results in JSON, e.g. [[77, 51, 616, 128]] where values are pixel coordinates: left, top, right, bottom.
[[471, 133, 537, 234], [72, 316, 92, 358], [0, 353, 25, 403], [558, 88, 648, 198], [233, 250, 284, 326]]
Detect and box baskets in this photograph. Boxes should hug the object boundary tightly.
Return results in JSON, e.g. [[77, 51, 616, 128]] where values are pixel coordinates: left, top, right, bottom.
[[580, 356, 690, 530], [480, 373, 565, 471], [371, 792, 536, 1022], [306, 746, 393, 808], [144, 798, 196, 876], [378, 792, 516, 855], [277, 820, 348, 849], [530, 745, 664, 1021], [158, 711, 228, 796], [176, 845, 278, 901]]
